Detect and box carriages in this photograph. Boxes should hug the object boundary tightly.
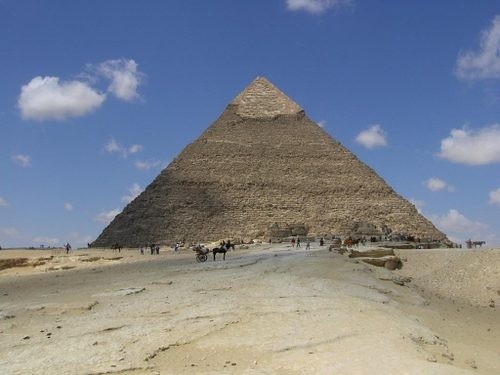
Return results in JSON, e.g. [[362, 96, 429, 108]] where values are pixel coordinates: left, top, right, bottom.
[[192, 243, 235, 263]]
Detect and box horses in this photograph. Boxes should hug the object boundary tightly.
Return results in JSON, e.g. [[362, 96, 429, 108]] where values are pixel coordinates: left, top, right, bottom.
[[473, 241, 485, 248], [212, 243, 230, 260], [348, 238, 362, 247]]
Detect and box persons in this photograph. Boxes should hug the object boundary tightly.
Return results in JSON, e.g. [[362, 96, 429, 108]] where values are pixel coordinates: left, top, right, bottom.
[[200, 245, 207, 252], [221, 240, 226, 246], [113, 243, 180, 254], [345, 235, 463, 249], [87, 242, 91, 248], [291, 238, 323, 251], [65, 242, 72, 253]]
